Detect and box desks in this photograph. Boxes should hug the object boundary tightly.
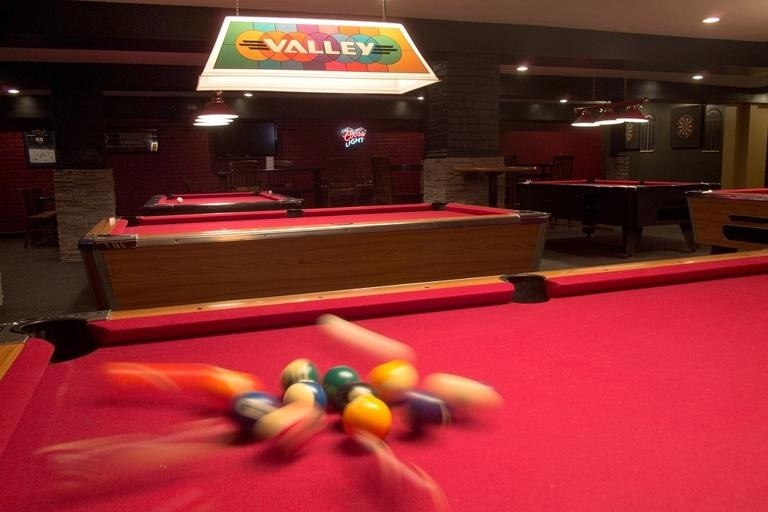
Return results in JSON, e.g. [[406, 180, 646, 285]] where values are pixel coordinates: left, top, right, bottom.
[[454, 163, 538, 208]]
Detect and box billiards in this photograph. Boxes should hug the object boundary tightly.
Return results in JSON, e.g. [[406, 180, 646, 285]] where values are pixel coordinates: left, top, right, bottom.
[[230, 359, 502, 454], [176, 197, 183, 203]]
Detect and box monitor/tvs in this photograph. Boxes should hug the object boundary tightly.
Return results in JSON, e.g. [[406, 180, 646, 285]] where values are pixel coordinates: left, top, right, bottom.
[[215, 117, 278, 159]]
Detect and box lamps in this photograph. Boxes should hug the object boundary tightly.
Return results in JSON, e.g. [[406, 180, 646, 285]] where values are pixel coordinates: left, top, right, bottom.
[[568, 69, 651, 128], [191, 91, 239, 127]]
